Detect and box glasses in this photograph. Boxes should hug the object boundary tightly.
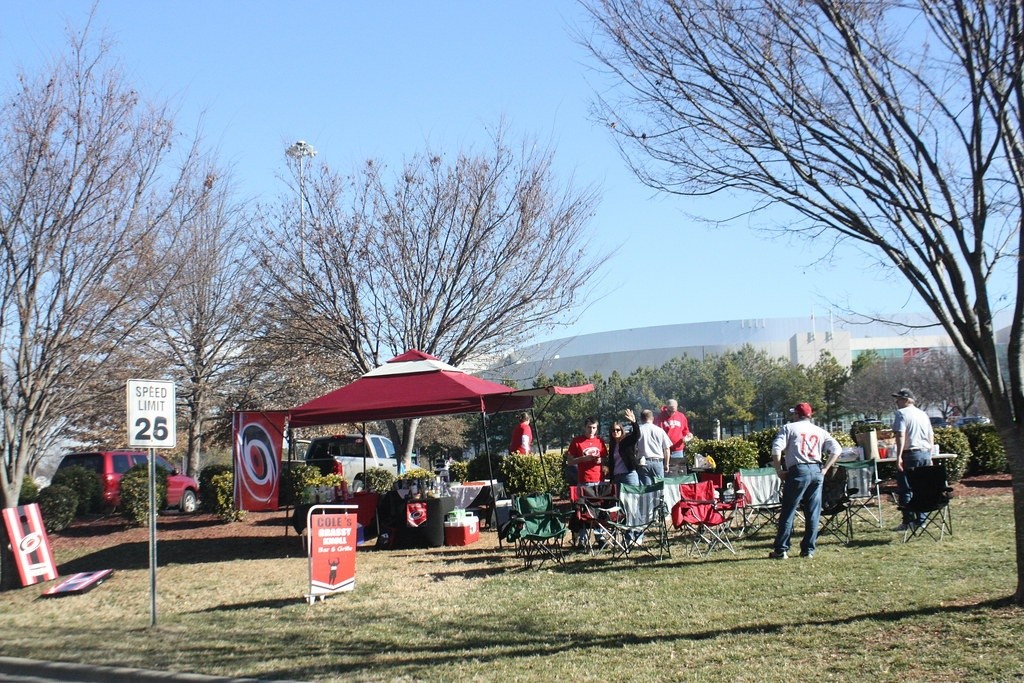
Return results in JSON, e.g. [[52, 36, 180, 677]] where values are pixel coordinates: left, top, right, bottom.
[[612, 428, 620, 432]]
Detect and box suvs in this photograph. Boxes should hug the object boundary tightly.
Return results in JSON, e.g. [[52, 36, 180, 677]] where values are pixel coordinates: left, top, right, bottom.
[[51, 452, 199, 515]]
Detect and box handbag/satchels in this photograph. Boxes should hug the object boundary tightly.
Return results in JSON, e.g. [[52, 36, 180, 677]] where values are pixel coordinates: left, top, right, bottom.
[[856, 426, 880, 460]]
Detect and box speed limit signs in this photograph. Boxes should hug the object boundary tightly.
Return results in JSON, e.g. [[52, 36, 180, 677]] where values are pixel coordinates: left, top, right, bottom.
[[126, 378, 176, 450]]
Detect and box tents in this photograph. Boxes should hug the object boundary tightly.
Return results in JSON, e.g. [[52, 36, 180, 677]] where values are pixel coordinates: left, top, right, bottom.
[[285, 349, 595, 552]]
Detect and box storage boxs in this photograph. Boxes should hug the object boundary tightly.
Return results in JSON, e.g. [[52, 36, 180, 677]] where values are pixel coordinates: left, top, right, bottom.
[[496, 499, 513, 528], [445, 515, 479, 545]]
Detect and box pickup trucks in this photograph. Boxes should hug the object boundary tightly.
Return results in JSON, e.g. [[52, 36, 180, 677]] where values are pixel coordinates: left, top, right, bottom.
[[279, 434, 421, 505]]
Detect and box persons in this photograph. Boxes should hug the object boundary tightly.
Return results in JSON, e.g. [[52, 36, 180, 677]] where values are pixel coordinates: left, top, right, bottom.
[[508, 412, 534, 456], [771, 403, 841, 560], [568, 399, 692, 554], [891, 389, 934, 532]]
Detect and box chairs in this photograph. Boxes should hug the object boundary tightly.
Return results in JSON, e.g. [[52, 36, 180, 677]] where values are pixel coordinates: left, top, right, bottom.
[[498, 454, 957, 571]]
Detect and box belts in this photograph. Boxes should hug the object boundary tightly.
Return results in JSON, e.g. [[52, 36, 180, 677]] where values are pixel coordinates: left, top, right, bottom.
[[903, 449, 919, 453]]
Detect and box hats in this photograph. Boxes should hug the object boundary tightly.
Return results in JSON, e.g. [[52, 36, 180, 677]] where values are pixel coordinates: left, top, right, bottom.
[[790, 403, 811, 417], [892, 388, 915, 400]]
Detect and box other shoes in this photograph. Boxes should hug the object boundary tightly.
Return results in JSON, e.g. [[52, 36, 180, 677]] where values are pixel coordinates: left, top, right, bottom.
[[890, 522, 909, 531], [800, 551, 813, 559], [630, 540, 642, 546], [915, 523, 926, 529], [770, 550, 788, 560]]
